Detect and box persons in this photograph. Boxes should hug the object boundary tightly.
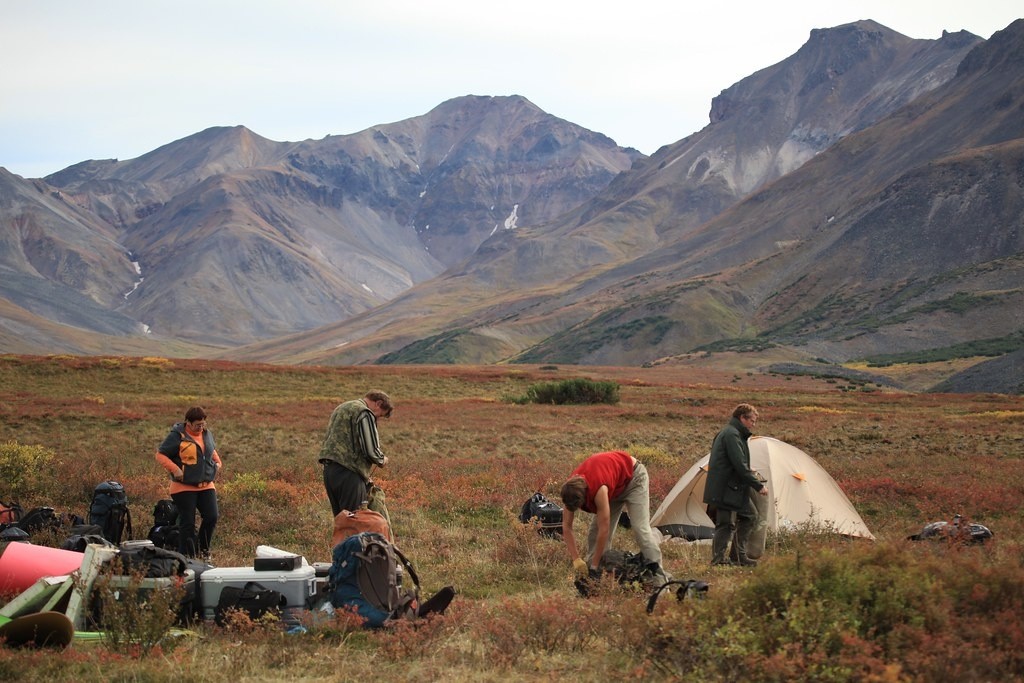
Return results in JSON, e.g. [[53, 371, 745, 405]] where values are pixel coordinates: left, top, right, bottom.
[[560, 452, 672, 582], [318, 390, 393, 516], [155, 406, 222, 557], [703, 403, 768, 567]]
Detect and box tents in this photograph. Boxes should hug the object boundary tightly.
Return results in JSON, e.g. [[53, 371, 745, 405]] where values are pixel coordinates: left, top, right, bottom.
[[650, 436, 876, 544]]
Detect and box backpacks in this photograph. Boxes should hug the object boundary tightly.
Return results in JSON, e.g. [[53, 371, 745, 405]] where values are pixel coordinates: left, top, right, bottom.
[[321, 531, 420, 627], [87, 481, 132, 547], [153, 499, 181, 529]]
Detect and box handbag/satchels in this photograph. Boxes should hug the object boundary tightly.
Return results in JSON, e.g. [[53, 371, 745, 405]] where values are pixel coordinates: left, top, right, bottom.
[[215, 580, 286, 629]]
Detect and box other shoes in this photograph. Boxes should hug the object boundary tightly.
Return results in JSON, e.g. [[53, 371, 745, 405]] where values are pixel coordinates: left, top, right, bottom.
[[735, 558, 754, 566]]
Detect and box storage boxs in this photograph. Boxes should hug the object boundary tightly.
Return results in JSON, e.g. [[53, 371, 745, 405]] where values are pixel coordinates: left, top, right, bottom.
[[93, 567, 195, 629], [312, 562, 402, 601], [199, 564, 317, 626]]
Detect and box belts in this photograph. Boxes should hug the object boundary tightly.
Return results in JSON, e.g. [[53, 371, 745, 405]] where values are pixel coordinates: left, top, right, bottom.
[[632, 461, 640, 471]]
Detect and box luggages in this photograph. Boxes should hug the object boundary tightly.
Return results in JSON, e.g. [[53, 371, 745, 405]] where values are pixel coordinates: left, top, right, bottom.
[[18, 506, 55, 530]]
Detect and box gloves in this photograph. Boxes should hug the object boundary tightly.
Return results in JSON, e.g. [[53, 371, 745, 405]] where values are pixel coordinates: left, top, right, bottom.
[[572, 558, 589, 577]]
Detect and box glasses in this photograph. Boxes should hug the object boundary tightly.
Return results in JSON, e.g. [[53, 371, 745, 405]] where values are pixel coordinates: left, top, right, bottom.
[[191, 423, 206, 428]]
